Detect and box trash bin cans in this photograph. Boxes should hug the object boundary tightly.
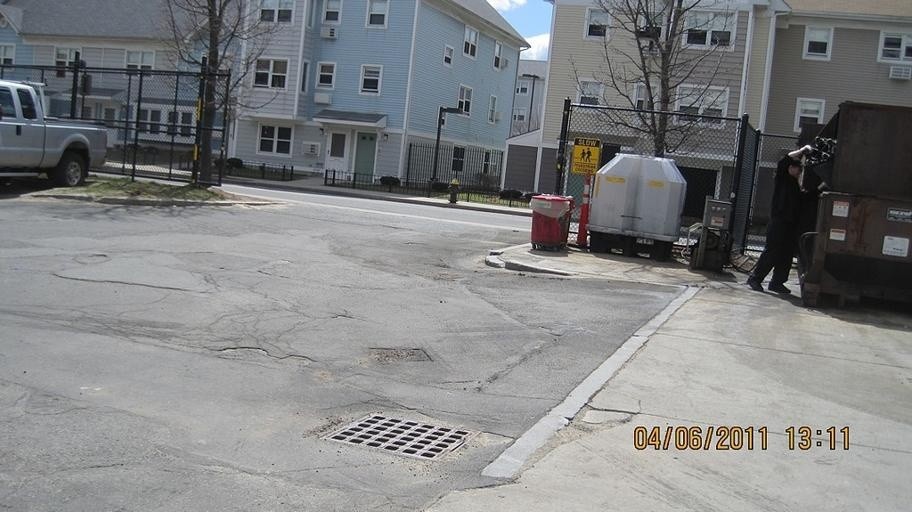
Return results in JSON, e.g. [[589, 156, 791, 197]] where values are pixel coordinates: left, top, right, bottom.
[[529, 194, 576, 252]]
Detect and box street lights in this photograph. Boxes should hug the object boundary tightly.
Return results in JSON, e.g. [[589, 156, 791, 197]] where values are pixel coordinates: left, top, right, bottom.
[[432, 107, 464, 183]]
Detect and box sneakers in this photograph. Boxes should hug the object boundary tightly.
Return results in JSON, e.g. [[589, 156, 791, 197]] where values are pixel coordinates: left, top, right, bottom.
[[768, 284, 791, 293], [748, 276, 763, 292]]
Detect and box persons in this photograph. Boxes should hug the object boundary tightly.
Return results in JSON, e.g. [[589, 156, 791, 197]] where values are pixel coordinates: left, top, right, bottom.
[[585, 147, 591, 162], [580, 148, 586, 160], [746, 143, 830, 292]]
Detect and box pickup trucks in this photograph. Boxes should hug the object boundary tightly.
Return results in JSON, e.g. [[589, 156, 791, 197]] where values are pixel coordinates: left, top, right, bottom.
[[0, 77, 110, 188]]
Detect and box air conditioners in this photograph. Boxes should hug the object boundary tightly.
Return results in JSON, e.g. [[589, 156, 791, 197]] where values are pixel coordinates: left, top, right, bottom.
[[319, 25, 338, 39], [502, 56, 513, 71], [302, 141, 320, 154], [313, 93, 333, 105], [888, 65, 912, 79], [494, 111, 507, 121]]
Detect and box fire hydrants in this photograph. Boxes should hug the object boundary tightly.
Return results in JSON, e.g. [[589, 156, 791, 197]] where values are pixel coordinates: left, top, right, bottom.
[[446, 178, 460, 204]]
